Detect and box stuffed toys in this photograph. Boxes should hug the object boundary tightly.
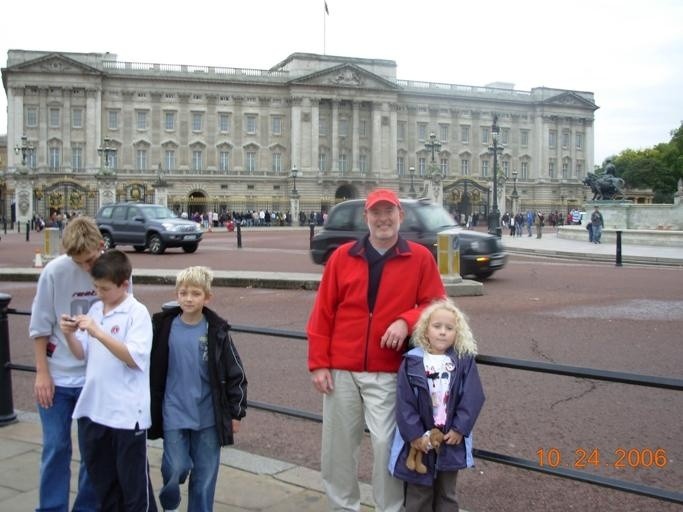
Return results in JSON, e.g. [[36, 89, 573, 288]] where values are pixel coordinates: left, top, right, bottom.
[[404, 428, 445, 475]]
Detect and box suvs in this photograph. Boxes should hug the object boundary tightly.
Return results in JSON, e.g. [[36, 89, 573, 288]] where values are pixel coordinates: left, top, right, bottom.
[[92, 203, 206, 255], [307, 194, 513, 284]]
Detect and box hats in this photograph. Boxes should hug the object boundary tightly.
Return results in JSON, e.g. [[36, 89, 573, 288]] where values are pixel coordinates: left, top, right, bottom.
[[365, 188, 401, 212]]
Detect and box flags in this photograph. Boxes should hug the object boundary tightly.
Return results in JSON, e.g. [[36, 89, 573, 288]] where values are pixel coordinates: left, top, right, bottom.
[[322, 0, 330, 16]]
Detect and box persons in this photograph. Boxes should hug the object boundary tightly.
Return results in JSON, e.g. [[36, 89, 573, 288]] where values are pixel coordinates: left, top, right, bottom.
[[590, 203, 604, 244], [586, 222, 592, 242], [25, 214, 134, 512], [149, 263, 247, 512], [59, 246, 159, 511], [447, 206, 585, 240], [384, 293, 487, 512], [303, 186, 449, 512], [28, 207, 83, 232], [172, 204, 328, 229], [598, 156, 618, 178]]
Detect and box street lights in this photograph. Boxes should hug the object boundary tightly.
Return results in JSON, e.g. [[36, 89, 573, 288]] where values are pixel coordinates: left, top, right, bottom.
[[512, 168, 519, 195], [406, 165, 418, 201], [96, 135, 118, 176], [288, 165, 299, 195], [14, 133, 36, 176], [483, 115, 508, 239], [423, 131, 444, 176]]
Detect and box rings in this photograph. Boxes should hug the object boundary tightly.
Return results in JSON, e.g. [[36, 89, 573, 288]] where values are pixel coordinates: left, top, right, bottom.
[[391, 340, 397, 344]]
